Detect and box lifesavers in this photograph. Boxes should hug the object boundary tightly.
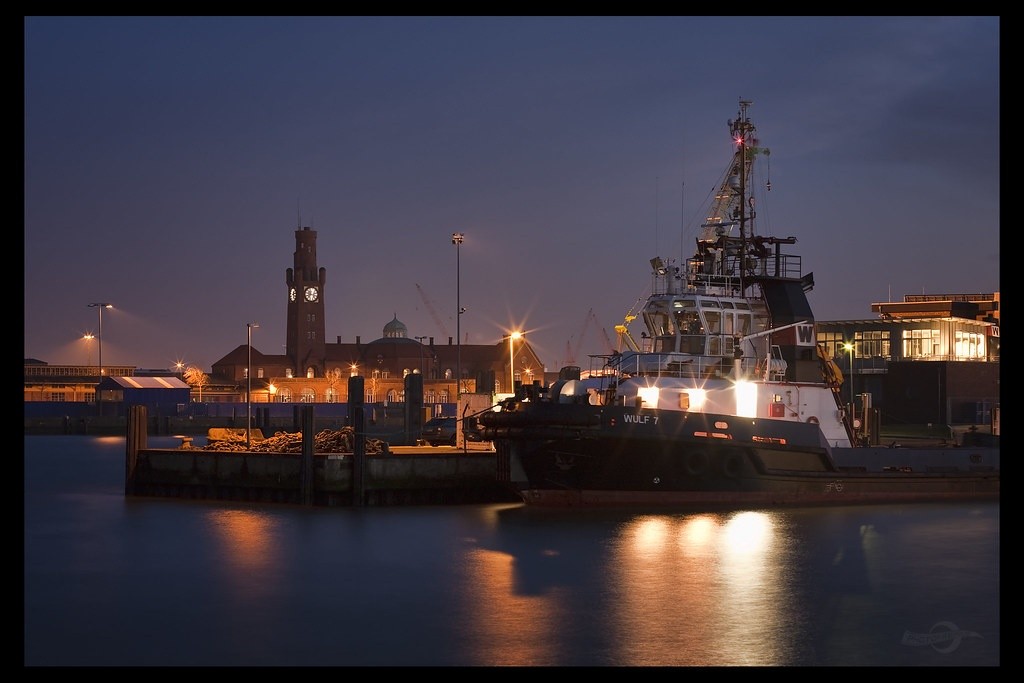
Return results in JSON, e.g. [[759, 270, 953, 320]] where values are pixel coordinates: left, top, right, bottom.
[[807, 415, 820, 426]]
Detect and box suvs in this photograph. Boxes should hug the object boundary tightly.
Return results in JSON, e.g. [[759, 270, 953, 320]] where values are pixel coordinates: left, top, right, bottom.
[[422, 416, 458, 447]]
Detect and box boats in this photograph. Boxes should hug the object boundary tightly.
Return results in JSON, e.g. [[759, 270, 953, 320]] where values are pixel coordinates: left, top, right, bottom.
[[458, 98, 1001, 508]]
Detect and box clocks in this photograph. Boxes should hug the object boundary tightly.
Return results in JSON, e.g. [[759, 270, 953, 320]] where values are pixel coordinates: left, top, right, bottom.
[[304, 286, 318, 302], [290, 287, 296, 302]]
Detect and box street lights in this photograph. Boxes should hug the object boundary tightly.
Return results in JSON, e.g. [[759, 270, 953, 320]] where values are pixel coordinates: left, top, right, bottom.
[[246, 324, 260, 449], [87, 303, 112, 416], [84, 334, 95, 366], [510, 332, 521, 393], [414, 336, 428, 375], [842, 343, 855, 435]]
